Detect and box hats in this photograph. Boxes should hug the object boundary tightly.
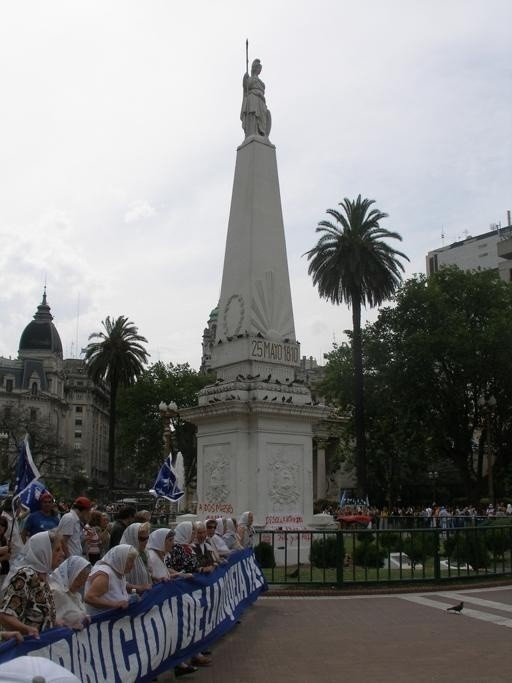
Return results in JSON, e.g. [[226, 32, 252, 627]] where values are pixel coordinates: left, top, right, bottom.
[[77, 497, 91, 508]]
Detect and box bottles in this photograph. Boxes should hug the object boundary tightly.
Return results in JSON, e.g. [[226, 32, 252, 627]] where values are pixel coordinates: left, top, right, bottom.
[[128, 587, 138, 604]]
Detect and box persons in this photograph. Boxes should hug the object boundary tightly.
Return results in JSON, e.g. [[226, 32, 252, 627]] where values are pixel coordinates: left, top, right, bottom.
[[240, 58, 267, 138], [312, 498, 512, 537], [0, 489, 254, 683]]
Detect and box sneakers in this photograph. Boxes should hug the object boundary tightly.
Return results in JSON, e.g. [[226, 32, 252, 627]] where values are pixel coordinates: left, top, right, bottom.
[[175, 664, 197, 676], [191, 655, 212, 666]]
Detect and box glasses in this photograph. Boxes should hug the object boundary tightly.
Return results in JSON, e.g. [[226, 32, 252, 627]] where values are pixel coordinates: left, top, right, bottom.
[[139, 536, 149, 542], [207, 525, 217, 529], [44, 501, 52, 504]]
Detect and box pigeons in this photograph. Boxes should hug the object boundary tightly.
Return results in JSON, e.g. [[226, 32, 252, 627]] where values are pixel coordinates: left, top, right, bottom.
[[215, 394, 297, 403], [212, 373, 308, 388], [285, 569, 300, 579], [446, 602, 465, 614]]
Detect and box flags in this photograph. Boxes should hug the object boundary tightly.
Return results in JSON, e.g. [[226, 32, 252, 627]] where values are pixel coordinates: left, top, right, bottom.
[[11, 440, 55, 518], [149, 455, 185, 503]]
